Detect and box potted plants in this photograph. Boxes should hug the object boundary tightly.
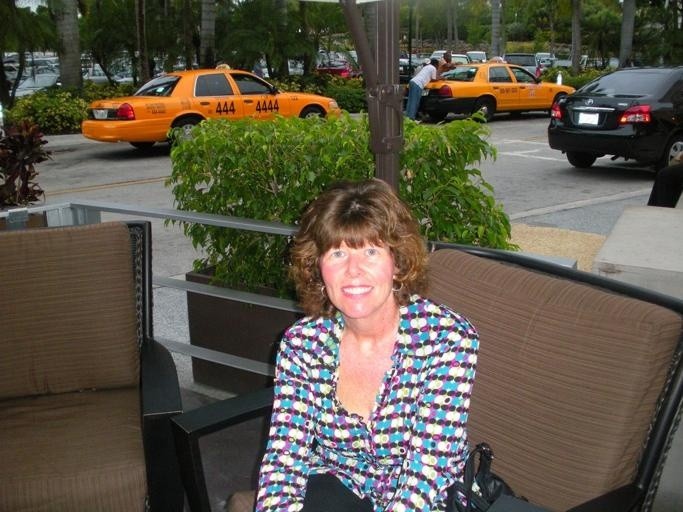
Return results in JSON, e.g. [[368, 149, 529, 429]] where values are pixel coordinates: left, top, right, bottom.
[[170, 115, 514, 394]]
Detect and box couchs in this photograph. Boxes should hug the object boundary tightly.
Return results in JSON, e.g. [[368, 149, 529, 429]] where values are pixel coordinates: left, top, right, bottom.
[[3, 217, 182, 512], [171, 246, 683, 512]]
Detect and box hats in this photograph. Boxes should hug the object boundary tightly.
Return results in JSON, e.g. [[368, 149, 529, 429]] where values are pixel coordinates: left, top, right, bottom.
[[421, 58, 430, 64]]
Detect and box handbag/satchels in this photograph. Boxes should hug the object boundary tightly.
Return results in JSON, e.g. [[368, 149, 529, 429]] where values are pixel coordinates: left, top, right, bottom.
[[445, 442, 528, 512]]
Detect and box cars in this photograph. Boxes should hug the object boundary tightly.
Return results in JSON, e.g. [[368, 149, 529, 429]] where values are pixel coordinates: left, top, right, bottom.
[[252, 50, 363, 80], [399, 51, 577, 123], [81, 63, 341, 147], [579, 55, 619, 71], [548, 66, 683, 172], [3, 52, 140, 96]]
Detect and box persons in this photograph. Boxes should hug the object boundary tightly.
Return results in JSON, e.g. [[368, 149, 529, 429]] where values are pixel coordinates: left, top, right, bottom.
[[414, 58, 431, 77], [406, 58, 444, 120], [254, 177, 480, 512], [437, 52, 456, 73]]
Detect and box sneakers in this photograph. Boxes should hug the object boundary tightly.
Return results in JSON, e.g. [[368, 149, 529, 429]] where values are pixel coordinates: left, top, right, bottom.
[[414, 119, 420, 124]]
